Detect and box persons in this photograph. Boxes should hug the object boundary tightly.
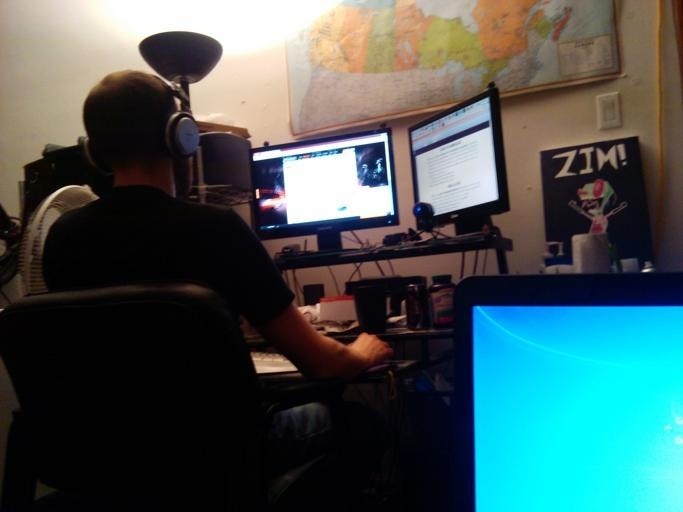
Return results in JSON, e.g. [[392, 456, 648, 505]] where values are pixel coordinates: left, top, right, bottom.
[[41, 70, 396, 511]]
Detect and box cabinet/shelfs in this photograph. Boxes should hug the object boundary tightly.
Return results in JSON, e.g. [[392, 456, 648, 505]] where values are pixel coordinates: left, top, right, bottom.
[[274, 236, 513, 346]]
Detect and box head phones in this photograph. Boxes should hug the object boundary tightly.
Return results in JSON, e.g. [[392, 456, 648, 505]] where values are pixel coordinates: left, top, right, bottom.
[[77, 71, 202, 160]]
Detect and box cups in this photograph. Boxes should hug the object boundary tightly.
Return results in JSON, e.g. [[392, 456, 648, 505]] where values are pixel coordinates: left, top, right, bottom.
[[572, 233, 609, 275], [303, 284, 325, 305]]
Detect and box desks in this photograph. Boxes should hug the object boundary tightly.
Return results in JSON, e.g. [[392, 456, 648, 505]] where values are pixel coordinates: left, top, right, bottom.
[[257, 359, 420, 502]]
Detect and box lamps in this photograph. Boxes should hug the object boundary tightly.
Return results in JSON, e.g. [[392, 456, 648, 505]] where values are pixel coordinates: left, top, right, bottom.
[[139, 32, 222, 114]]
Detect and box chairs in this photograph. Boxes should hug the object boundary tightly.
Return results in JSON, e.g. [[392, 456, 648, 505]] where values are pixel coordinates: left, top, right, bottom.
[[0, 282, 345, 512]]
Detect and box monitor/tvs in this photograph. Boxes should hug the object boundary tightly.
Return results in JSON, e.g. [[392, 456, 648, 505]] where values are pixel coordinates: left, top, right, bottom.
[[452, 272, 683, 512], [407, 88, 509, 237], [247, 127, 400, 269]]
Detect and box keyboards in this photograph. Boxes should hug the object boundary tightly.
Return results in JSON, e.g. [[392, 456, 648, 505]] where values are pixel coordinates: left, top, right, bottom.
[[250, 349, 298, 374]]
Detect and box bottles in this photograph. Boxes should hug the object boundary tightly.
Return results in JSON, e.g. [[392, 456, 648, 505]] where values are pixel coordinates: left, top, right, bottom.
[[406, 284, 423, 330], [429, 274, 458, 330]]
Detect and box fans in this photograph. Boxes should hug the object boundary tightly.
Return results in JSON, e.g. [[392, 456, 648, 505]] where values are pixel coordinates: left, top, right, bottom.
[[19, 184, 100, 293]]
